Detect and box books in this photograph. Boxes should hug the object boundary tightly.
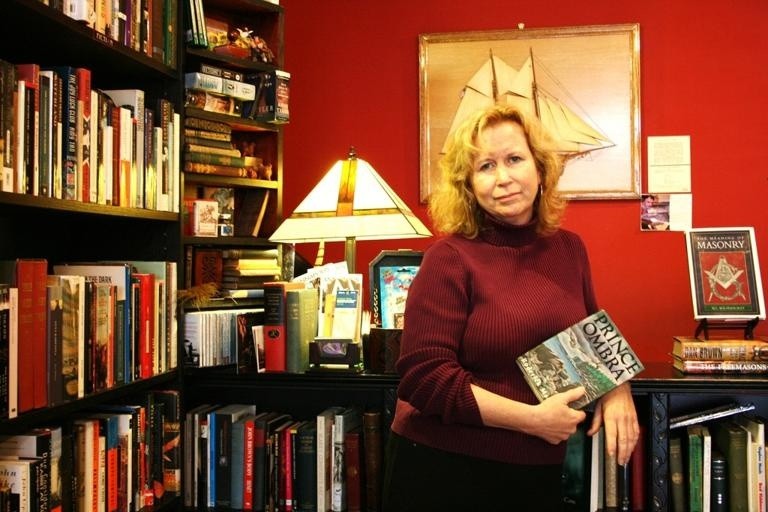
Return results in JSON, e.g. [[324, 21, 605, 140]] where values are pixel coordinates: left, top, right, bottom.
[[1, 248, 420, 511], [563, 400, 768, 512], [1, 2, 289, 237], [656, 222, 669, 231], [667, 335, 768, 374], [516, 309, 645, 411]]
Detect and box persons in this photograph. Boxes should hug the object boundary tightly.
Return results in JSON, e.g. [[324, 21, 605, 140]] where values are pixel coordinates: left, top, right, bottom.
[[641, 195, 664, 230], [382, 104, 640, 510]]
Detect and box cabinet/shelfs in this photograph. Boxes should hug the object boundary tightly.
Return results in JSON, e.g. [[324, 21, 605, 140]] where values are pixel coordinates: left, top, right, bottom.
[[187, 0, 287, 251], [181, 371, 768, 512], [0, 2, 186, 512]]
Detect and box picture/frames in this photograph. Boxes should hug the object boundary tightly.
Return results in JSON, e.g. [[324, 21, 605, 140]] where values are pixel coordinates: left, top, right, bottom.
[[418, 23, 641, 205]]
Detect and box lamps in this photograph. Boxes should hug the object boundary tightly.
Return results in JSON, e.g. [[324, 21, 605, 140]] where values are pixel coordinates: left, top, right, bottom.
[[267, 145, 434, 273]]
